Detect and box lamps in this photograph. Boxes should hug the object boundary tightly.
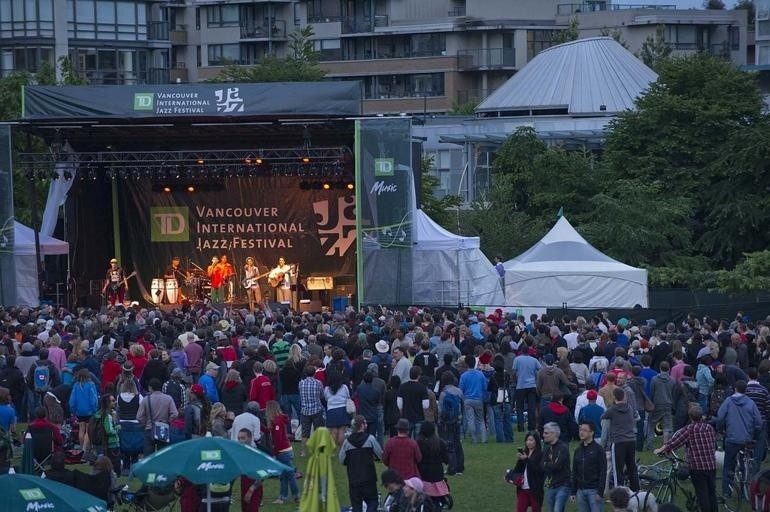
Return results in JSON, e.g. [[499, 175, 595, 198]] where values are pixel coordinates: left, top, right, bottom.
[[37, 168, 48, 180], [309, 166, 318, 177], [284, 166, 294, 174], [272, 165, 282, 175], [63, 168, 73, 181], [183, 167, 194, 178], [150, 182, 227, 193], [335, 164, 345, 177], [297, 165, 306, 176], [76, 169, 85, 181], [88, 168, 97, 181], [303, 153, 310, 162], [222, 167, 232, 178], [247, 166, 256, 177], [198, 156, 204, 163], [157, 167, 167, 180], [255, 156, 262, 164], [198, 167, 209, 178], [245, 154, 252, 163], [142, 168, 152, 181], [50, 169, 61, 181], [235, 166, 244, 177], [117, 167, 130, 180], [169, 167, 181, 179], [105, 168, 116, 181], [321, 164, 331, 176], [211, 167, 220, 178], [131, 167, 141, 181], [25, 169, 34, 180], [298, 181, 354, 192]]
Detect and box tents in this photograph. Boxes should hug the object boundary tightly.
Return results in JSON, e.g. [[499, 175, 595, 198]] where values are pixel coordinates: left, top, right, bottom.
[[493, 207, 650, 307], [359, 208, 504, 305]]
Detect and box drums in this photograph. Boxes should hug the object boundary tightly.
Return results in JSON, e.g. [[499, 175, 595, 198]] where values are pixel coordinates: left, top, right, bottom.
[[166, 279, 178, 303], [151, 279, 165, 303]]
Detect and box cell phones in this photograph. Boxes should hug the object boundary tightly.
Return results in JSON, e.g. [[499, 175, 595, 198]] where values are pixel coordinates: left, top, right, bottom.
[[517, 448, 524, 455]]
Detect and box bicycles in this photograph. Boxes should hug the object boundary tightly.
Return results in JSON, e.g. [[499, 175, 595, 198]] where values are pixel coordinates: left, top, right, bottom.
[[643, 443, 742, 512], [735, 432, 761, 502], [410, 477, 453, 510], [634, 456, 702, 483], [597, 459, 656, 497]]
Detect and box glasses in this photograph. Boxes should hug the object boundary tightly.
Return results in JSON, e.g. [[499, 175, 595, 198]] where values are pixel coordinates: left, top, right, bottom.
[[579, 428, 593, 432], [110, 399, 115, 402], [543, 431, 553, 434]]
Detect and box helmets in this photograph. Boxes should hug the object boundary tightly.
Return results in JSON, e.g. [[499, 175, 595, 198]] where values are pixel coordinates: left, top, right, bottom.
[[170, 368, 184, 379]]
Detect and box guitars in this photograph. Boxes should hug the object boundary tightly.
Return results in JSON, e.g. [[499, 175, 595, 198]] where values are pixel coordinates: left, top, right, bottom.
[[109, 270, 138, 296], [242, 272, 270, 288], [270, 267, 293, 288]]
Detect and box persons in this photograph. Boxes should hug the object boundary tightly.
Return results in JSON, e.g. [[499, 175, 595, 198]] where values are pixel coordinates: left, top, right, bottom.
[[207, 255, 226, 303], [164, 257, 183, 306], [2, 303, 768, 512], [102, 257, 128, 305], [37, 259, 47, 295], [268, 256, 299, 311], [493, 255, 505, 304], [220, 253, 235, 300], [244, 257, 262, 310]]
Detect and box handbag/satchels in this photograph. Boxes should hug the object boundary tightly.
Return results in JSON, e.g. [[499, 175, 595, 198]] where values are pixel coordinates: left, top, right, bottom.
[[559, 382, 573, 402], [645, 400, 656, 412], [342, 384, 357, 415], [504, 468, 524, 486], [150, 421, 171, 443], [492, 374, 512, 405], [689, 402, 700, 409]]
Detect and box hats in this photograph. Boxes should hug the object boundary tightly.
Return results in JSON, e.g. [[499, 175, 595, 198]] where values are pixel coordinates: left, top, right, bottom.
[[190, 384, 205, 397], [660, 361, 670, 370], [586, 377, 595, 385], [79, 368, 91, 377], [479, 354, 491, 364], [491, 360, 504, 372], [618, 318, 629, 327], [247, 401, 259, 414], [206, 362, 221, 370], [110, 258, 118, 263], [404, 477, 424, 494], [218, 320, 230, 332], [380, 469, 400, 487], [559, 357, 570, 368], [587, 388, 597, 400], [375, 339, 390, 354], [544, 353, 554, 360], [138, 318, 146, 326], [245, 256, 255, 263], [122, 361, 134, 371], [688, 406, 704, 417], [22, 342, 33, 352], [394, 418, 412, 431], [629, 326, 640, 334], [646, 319, 657, 325], [213, 331, 223, 337], [44, 319, 54, 331], [640, 339, 649, 348], [615, 356, 624, 365], [496, 308, 503, 315]]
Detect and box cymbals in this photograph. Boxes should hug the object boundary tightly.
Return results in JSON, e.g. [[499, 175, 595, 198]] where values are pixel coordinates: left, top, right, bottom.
[[191, 268, 202, 272]]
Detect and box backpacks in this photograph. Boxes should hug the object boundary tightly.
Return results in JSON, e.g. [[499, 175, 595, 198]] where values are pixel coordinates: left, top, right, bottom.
[[33, 361, 52, 393], [166, 380, 183, 410], [376, 353, 392, 383], [709, 388, 727, 417], [441, 390, 465, 426], [87, 413, 111, 447]]
